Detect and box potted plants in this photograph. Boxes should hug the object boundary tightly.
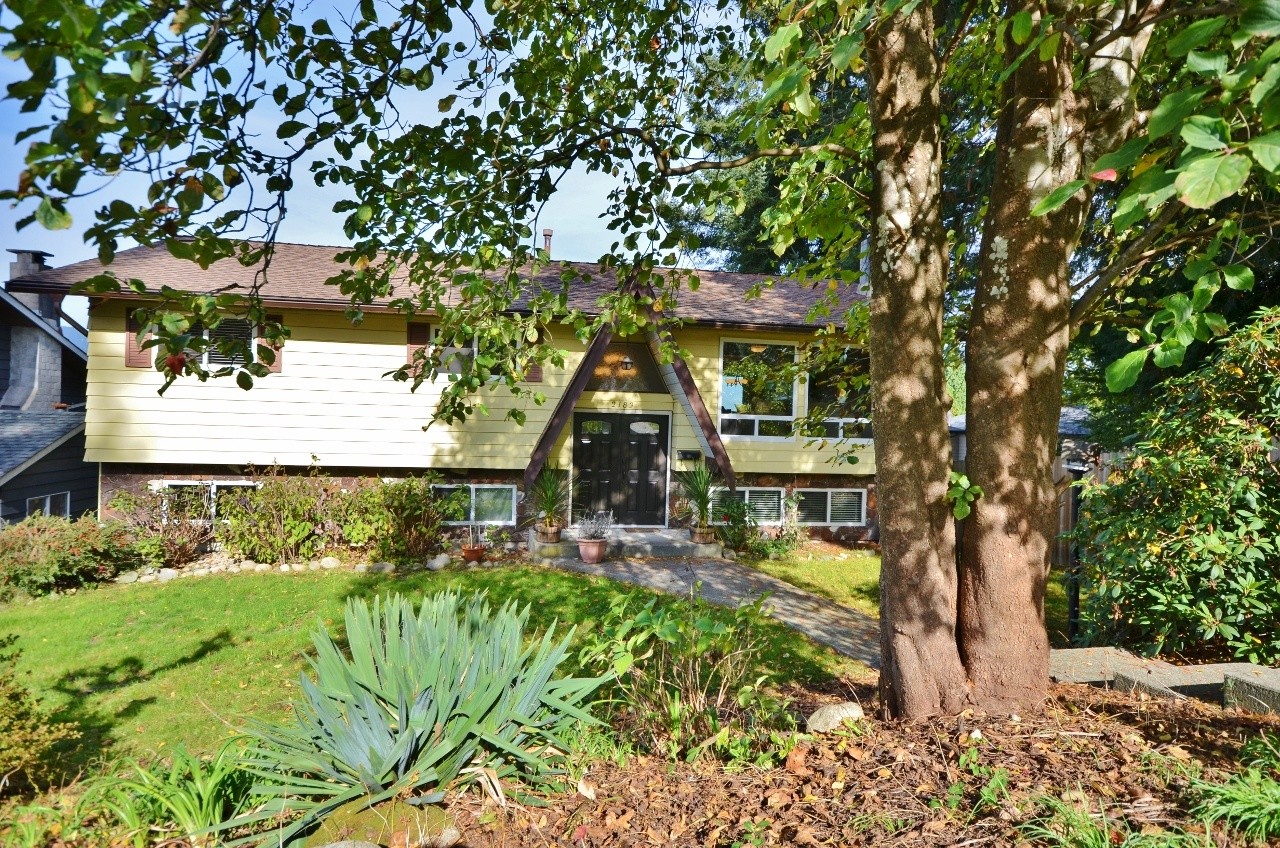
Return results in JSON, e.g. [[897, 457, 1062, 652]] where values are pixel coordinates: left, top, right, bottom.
[[673, 458, 730, 544], [526, 455, 581, 543]]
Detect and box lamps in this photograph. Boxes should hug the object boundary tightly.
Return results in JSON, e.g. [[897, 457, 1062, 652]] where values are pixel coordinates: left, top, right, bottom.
[[620, 334, 634, 370], [750, 344, 768, 353], [726, 375, 749, 387]]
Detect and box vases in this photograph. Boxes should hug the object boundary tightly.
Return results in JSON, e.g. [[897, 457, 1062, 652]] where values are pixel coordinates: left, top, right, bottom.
[[462, 543, 486, 564], [670, 509, 695, 528], [576, 540, 608, 564]]
